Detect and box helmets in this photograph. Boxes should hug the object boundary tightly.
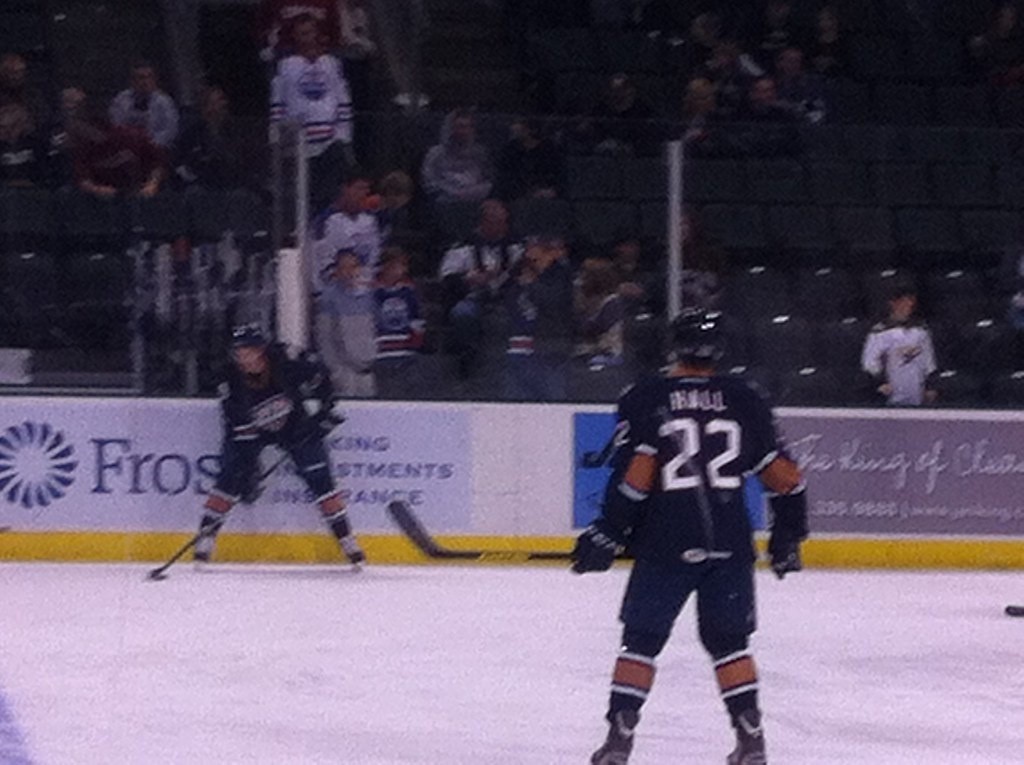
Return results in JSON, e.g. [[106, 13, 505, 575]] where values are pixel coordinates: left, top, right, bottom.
[[667, 307, 729, 359], [225, 320, 265, 349]]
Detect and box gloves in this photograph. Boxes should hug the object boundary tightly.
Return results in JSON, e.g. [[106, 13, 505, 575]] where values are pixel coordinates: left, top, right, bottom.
[[303, 396, 345, 440], [763, 479, 812, 579], [231, 456, 265, 504], [567, 480, 649, 574]]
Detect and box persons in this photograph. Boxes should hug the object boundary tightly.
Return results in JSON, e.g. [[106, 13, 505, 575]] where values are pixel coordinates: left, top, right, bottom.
[[0, 2, 1023, 407], [571, 312, 810, 764], [191, 323, 366, 564]]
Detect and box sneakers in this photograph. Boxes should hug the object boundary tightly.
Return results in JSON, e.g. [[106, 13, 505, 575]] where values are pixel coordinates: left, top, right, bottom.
[[346, 551, 370, 572], [591, 710, 639, 765], [193, 550, 209, 570], [726, 709, 766, 765]]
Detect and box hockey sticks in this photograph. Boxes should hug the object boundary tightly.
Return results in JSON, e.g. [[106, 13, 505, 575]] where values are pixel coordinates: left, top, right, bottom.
[[148, 429, 298, 582], [386, 496, 773, 565], [1003, 603, 1024, 618]]
